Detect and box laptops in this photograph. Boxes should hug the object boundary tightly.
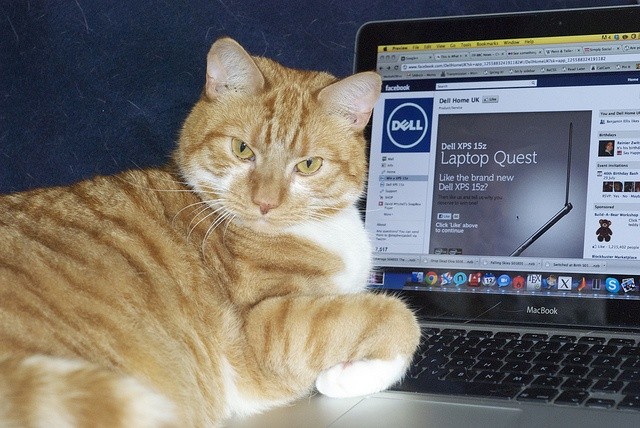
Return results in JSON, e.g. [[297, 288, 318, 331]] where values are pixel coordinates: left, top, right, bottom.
[[230, 2, 640, 427]]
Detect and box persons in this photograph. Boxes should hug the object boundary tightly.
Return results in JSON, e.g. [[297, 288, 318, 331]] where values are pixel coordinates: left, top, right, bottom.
[[602, 141, 614, 155]]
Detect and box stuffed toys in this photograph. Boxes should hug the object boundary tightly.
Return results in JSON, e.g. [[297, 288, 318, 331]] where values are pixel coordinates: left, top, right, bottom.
[[596, 219, 613, 241]]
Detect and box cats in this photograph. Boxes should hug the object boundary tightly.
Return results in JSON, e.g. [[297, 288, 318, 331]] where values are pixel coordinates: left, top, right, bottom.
[[0, 36, 422, 428]]
